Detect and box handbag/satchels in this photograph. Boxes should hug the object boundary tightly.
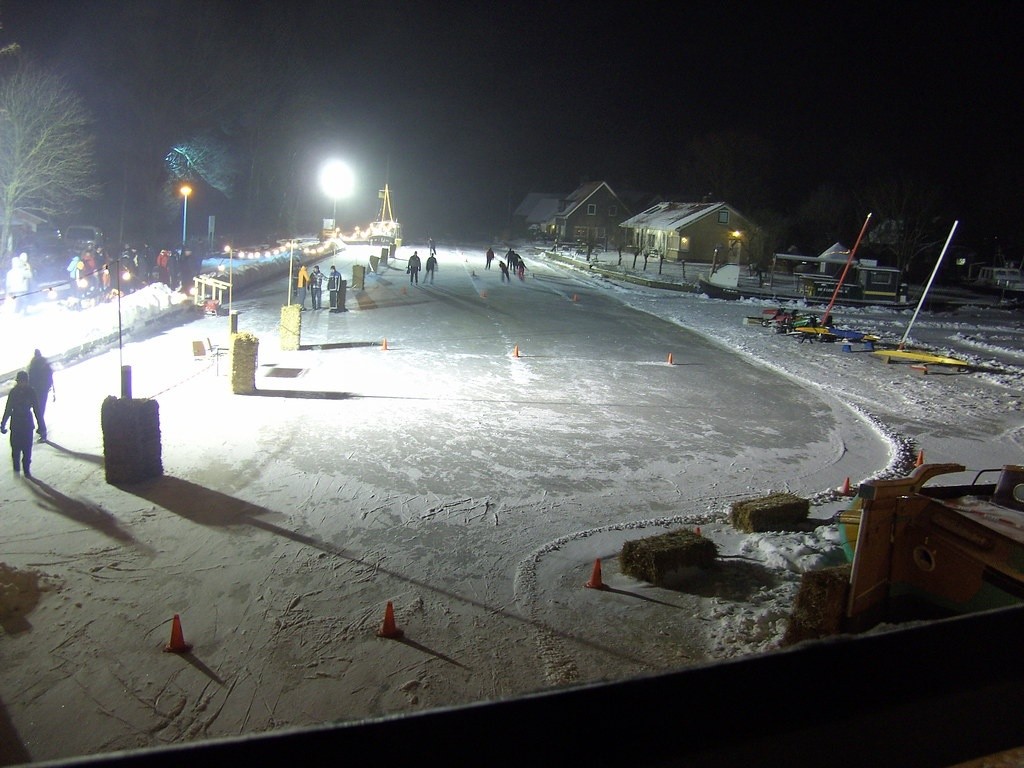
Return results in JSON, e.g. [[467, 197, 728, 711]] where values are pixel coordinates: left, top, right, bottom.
[[405, 270, 410, 274]]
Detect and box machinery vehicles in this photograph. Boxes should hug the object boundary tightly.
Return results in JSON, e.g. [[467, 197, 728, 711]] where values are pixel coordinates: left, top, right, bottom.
[[840, 459, 1023, 630]]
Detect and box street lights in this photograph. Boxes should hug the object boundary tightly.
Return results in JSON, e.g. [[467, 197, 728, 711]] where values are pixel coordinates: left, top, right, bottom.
[[318, 162, 356, 233], [224, 244, 233, 316], [179, 184, 193, 244]]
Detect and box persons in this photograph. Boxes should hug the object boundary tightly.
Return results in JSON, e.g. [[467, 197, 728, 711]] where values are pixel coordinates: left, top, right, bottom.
[[0, 371, 47, 474], [4, 242, 201, 315], [406, 251, 421, 285], [499, 247, 528, 283], [291, 255, 341, 311], [428, 236, 437, 255], [422, 253, 438, 284], [28, 349, 53, 444], [485, 248, 494, 269]]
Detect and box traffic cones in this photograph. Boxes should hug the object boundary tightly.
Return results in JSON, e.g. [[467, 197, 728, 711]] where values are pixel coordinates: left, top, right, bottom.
[[381, 338, 388, 350], [841, 477, 851, 495], [573, 293, 577, 301], [668, 352, 672, 364], [916, 451, 923, 464], [375, 601, 404, 639], [462, 252, 487, 298], [514, 345, 519, 357], [162, 614, 193, 652], [696, 528, 701, 535], [584, 559, 610, 590]]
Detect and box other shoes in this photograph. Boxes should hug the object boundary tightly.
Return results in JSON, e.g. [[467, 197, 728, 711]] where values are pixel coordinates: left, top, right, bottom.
[[312, 307, 321, 310], [329, 309, 337, 313], [301, 308, 306, 312]]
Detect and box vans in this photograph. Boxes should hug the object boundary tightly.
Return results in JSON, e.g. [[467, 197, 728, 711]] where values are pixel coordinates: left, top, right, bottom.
[[64, 224, 106, 249], [977, 267, 1021, 284]]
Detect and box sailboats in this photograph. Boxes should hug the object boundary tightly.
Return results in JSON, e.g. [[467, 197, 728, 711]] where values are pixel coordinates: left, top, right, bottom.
[[744, 215, 883, 353], [367, 184, 404, 245], [869, 219, 968, 374]]
